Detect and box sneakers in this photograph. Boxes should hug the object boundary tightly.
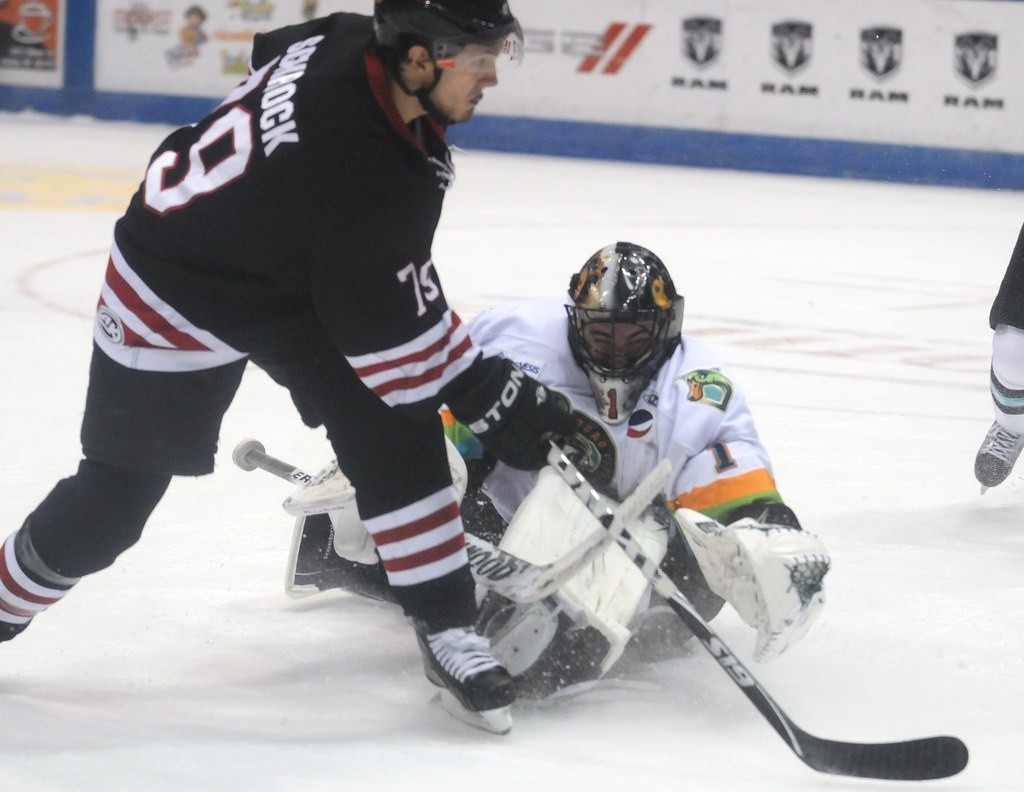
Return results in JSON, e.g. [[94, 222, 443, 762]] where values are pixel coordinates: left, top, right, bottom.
[[412, 618, 517, 735], [974, 421, 1024, 495]]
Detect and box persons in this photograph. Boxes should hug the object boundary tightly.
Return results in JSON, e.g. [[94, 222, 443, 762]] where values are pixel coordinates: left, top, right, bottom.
[[973, 221, 1024, 496], [0, 0, 575, 735], [284, 241, 824, 707]]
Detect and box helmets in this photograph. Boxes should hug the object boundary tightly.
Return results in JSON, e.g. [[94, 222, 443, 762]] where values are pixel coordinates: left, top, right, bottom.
[[563, 242, 685, 426], [373, 0, 525, 122]]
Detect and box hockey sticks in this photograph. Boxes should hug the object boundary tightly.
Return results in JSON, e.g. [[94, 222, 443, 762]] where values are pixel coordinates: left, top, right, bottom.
[[231, 437, 678, 608], [551, 442, 973, 781]]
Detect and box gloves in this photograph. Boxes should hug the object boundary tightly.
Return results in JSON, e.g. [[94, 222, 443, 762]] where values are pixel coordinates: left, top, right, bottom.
[[447, 350, 576, 472]]
